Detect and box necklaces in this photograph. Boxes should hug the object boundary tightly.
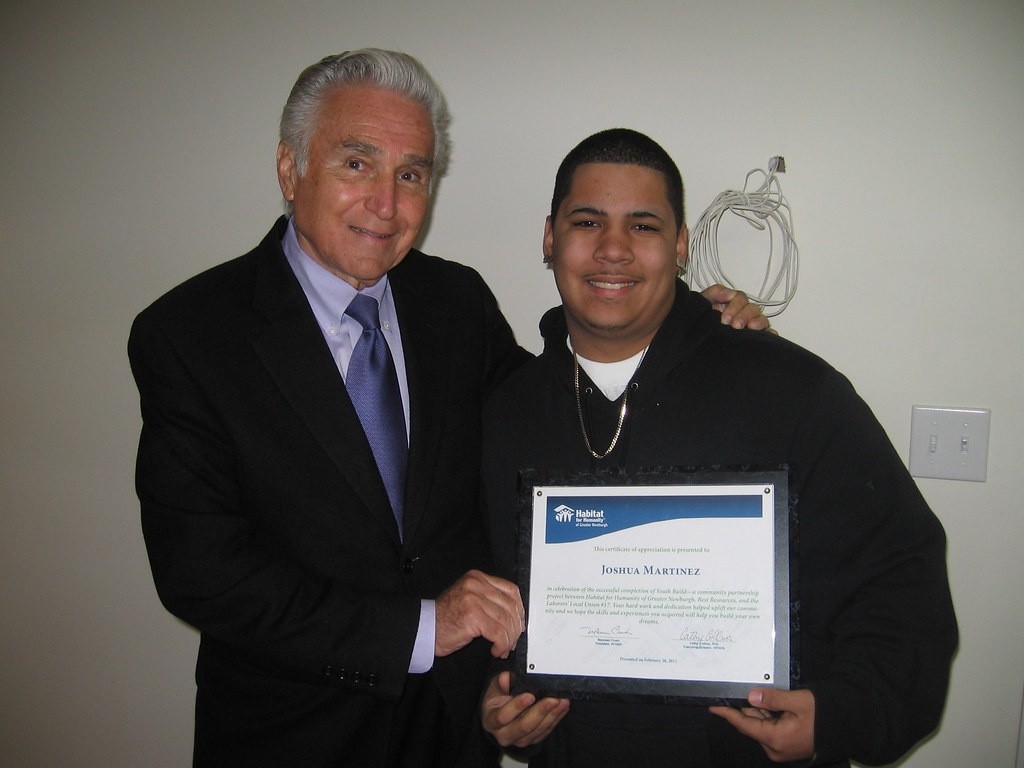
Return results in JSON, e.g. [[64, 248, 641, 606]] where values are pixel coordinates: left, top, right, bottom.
[[571, 344, 648, 459]]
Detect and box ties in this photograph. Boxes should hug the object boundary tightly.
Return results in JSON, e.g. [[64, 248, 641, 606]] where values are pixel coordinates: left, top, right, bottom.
[[343, 294, 409, 543]]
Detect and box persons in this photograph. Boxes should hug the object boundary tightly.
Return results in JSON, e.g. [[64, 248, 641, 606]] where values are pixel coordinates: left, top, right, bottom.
[[481, 127, 959, 768], [129, 48, 781, 768]]
